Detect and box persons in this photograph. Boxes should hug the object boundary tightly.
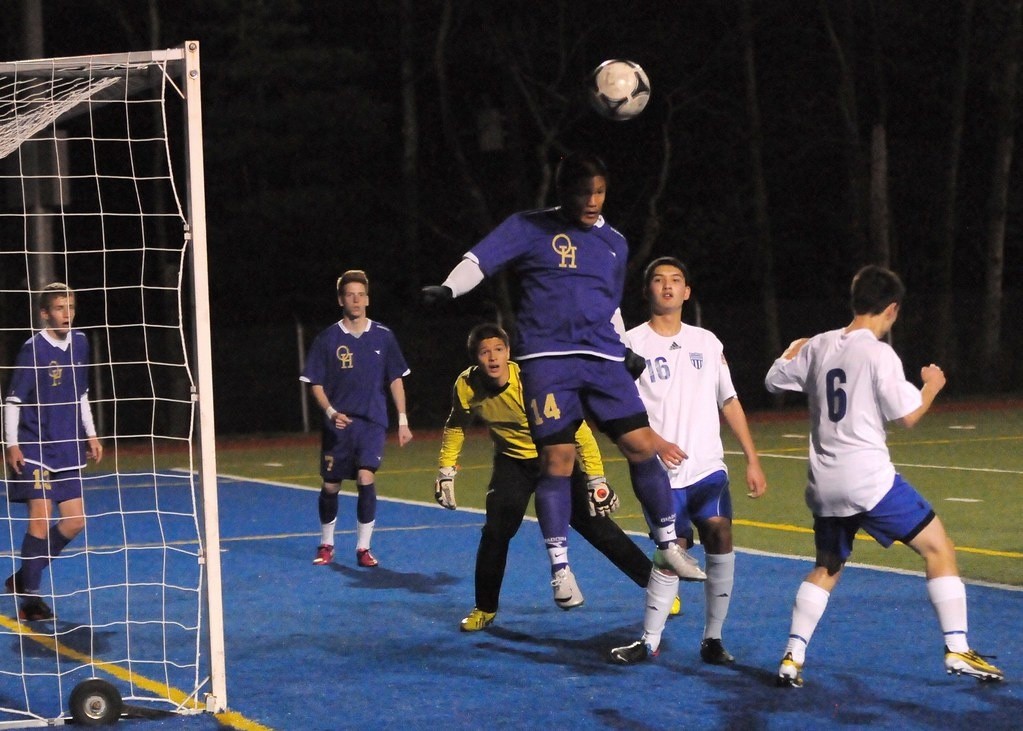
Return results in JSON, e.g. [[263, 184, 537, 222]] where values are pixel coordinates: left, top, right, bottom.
[[434, 326, 681, 632], [5, 282, 102, 622], [764, 266, 1004, 688], [298, 271, 412, 566], [611, 257, 767, 664], [421, 154, 707, 609]]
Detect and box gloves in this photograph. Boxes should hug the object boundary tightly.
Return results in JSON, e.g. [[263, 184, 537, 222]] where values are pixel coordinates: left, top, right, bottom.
[[433, 466, 458, 511], [420, 284, 453, 306], [623, 347, 647, 380], [586, 477, 619, 517]]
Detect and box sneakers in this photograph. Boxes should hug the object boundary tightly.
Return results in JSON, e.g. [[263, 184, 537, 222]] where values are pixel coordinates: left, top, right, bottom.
[[19, 603, 56, 621], [610, 640, 660, 664], [460, 607, 495, 632], [313, 544, 334, 565], [5, 574, 43, 606], [551, 564, 584, 608], [943, 643, 1004, 682], [357, 547, 378, 566], [670, 596, 681, 614], [700, 638, 734, 664], [778, 652, 803, 688], [653, 542, 708, 581]]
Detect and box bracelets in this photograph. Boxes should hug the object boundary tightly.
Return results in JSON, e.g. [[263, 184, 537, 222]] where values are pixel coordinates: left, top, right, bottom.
[[398, 413, 408, 425], [325, 406, 337, 420]]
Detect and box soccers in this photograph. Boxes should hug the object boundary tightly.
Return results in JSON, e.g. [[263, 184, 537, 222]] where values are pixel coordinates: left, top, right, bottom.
[[588, 59, 651, 120]]
